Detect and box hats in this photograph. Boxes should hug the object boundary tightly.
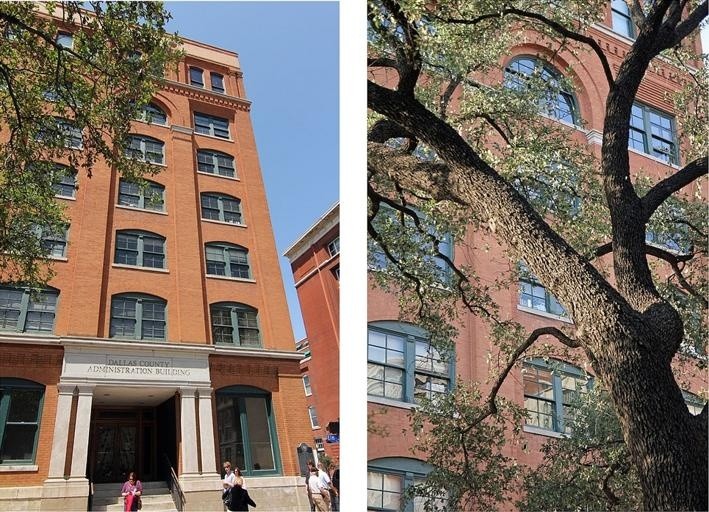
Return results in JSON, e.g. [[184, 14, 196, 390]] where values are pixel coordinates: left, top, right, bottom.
[[311, 467, 319, 472]]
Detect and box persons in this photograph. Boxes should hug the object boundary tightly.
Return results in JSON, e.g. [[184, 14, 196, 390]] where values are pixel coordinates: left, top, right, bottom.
[[222, 461, 256, 512], [305, 459, 338, 512], [121, 471, 143, 511]]
